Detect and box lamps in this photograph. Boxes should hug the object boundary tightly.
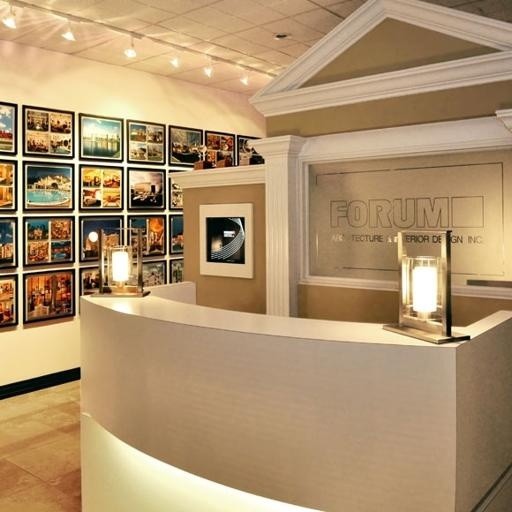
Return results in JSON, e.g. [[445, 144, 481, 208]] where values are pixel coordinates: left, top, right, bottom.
[[0, 0, 255, 88], [373, 225, 471, 346], [92, 245, 150, 297]]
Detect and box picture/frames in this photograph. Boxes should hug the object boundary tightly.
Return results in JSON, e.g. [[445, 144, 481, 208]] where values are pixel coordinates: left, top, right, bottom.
[[126, 167, 167, 210], [169, 258, 183, 284], [1, 217, 19, 269], [168, 214, 184, 257], [78, 214, 124, 263], [22, 215, 75, 268], [22, 160, 75, 211], [79, 266, 101, 317], [1, 160, 18, 211], [1, 274, 19, 327], [199, 203, 255, 280], [21, 104, 75, 161], [127, 214, 167, 259], [205, 130, 236, 169], [168, 124, 203, 168], [78, 163, 124, 210], [126, 119, 166, 166], [0, 101, 18, 156], [23, 268, 77, 324], [237, 134, 265, 167], [132, 260, 167, 287], [78, 112, 124, 163], [167, 169, 188, 212]]
